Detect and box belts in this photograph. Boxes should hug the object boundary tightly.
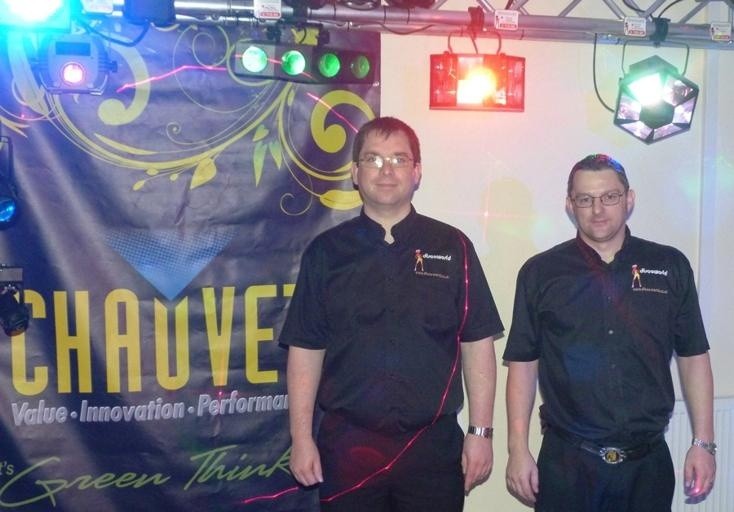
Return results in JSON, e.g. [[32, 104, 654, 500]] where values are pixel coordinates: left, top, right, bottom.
[[553, 428, 662, 465]]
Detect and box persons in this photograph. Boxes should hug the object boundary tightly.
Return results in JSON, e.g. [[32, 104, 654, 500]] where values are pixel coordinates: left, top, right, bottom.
[[503, 152, 719, 512], [274, 116, 505, 511]]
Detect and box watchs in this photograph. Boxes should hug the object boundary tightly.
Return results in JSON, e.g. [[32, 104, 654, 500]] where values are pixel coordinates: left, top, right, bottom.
[[468, 423, 495, 443], [692, 437, 719, 458]]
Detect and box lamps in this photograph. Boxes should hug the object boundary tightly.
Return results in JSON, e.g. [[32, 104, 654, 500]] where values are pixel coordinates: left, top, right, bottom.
[[429, 10, 527, 113], [607, 17, 700, 144], [0, 263, 31, 336], [234, 2, 378, 86], [38, 0, 113, 96], [0, 135, 20, 230]]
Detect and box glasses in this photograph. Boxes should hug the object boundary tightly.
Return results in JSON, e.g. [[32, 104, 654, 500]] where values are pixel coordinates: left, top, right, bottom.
[[358, 151, 414, 167], [571, 190, 625, 208]]
